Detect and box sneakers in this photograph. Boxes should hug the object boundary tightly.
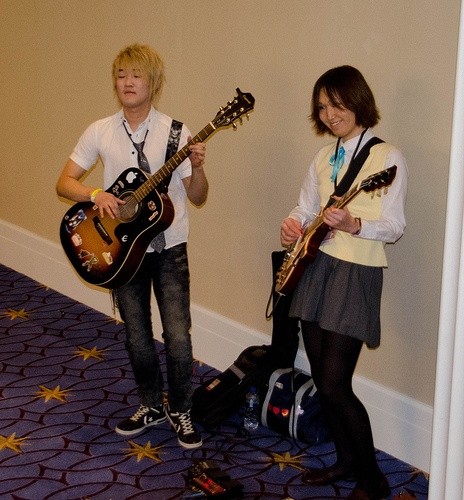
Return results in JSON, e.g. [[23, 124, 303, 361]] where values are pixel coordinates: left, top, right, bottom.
[[168, 409, 202, 449], [115, 404, 168, 436]]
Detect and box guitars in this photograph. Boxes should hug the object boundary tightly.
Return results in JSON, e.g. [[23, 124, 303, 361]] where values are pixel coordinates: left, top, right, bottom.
[[58, 87, 256, 290], [275, 165, 398, 297]]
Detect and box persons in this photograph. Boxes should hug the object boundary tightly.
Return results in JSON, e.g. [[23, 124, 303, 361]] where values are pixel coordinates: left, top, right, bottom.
[[57, 44, 209, 448], [280, 66, 410, 500]]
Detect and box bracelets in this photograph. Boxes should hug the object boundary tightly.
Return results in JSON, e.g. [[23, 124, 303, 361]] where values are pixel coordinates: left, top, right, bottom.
[[352, 217, 361, 236], [90, 189, 104, 202]]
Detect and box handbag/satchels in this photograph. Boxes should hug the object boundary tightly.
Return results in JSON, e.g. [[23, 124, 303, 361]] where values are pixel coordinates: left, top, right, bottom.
[[255, 366, 333, 446]]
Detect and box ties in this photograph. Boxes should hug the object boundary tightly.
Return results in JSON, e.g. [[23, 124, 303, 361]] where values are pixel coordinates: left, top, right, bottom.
[[122, 123, 167, 254]]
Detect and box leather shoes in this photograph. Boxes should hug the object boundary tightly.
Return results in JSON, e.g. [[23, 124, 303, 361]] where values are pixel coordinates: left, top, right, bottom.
[[344, 490, 391, 500], [300, 463, 356, 486]]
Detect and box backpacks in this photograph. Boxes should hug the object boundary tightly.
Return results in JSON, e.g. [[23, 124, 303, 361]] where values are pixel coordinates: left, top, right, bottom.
[[189, 344, 274, 436]]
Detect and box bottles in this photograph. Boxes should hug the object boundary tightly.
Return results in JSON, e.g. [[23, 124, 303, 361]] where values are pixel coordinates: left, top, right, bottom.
[[243, 386, 260, 431]]
